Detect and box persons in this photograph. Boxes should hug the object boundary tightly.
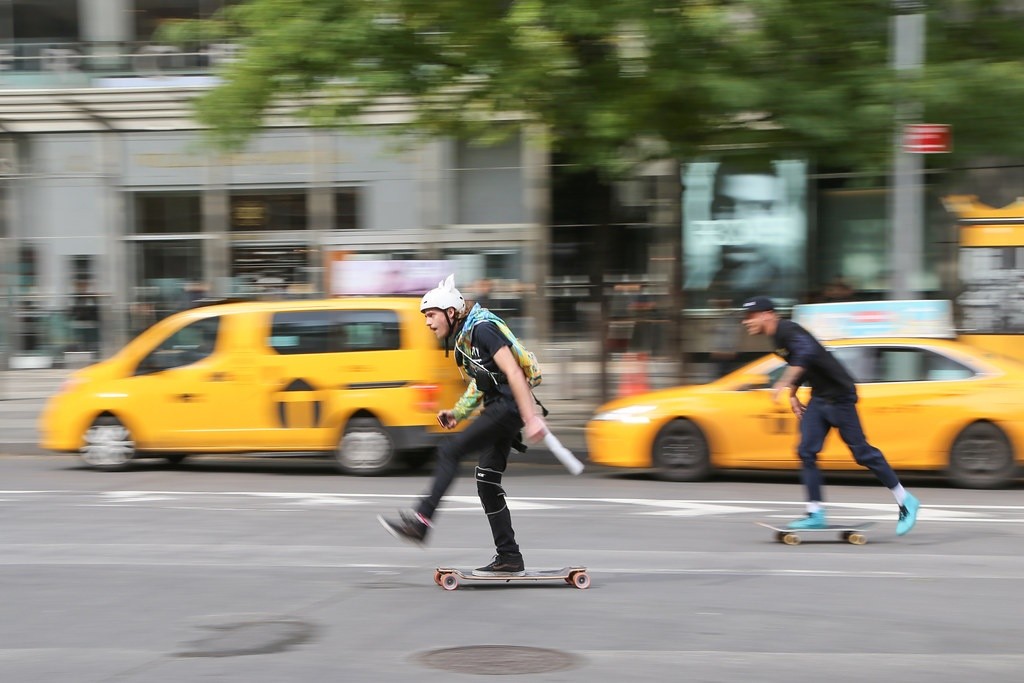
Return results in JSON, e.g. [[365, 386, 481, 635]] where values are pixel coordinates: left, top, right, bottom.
[[378, 274, 549, 578], [742, 297, 919, 536]]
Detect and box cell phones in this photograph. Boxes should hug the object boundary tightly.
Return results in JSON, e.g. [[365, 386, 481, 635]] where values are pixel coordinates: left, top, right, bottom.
[[438, 415, 448, 428]]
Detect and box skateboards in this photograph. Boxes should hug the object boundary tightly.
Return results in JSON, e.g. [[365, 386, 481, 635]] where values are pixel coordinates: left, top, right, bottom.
[[432, 563, 592, 591], [755, 520, 878, 545]]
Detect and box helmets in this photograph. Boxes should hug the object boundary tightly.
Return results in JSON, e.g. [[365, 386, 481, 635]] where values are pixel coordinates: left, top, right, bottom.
[[421, 273, 466, 314]]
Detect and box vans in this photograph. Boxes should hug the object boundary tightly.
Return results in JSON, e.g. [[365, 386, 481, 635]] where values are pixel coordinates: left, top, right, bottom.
[[37, 298, 476, 475]]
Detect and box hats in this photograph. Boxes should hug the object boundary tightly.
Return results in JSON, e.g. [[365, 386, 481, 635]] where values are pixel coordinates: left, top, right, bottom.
[[736, 298, 775, 314]]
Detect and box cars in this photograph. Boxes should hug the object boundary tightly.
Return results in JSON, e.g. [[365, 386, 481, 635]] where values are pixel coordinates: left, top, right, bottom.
[[586, 334, 1024, 488]]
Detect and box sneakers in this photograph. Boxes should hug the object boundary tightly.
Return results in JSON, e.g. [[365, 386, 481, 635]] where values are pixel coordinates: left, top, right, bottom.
[[895, 492, 919, 536], [786, 507, 828, 530], [376, 509, 432, 542], [472, 554, 525, 578]]
